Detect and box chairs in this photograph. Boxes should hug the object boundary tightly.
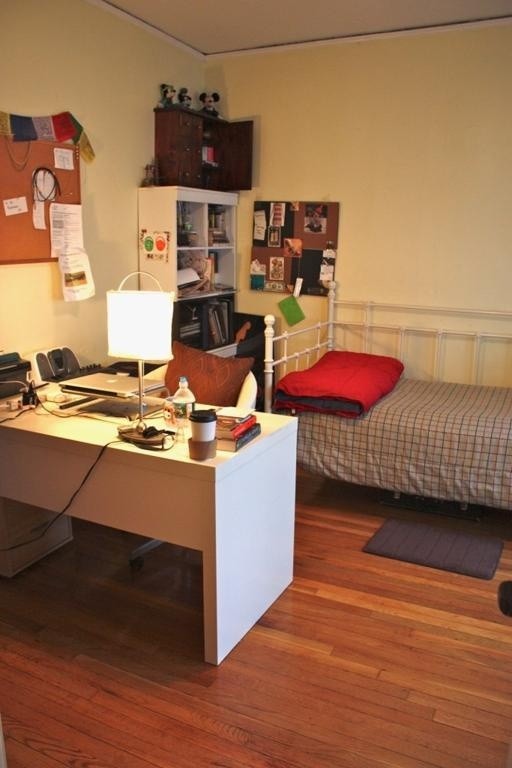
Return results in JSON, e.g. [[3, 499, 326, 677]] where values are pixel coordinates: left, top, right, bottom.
[[128, 363, 258, 567]]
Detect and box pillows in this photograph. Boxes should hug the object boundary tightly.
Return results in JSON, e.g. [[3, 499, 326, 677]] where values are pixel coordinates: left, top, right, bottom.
[[161, 339, 256, 408]]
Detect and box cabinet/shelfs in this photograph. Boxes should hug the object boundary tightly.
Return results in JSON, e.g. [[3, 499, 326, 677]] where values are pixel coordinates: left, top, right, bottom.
[[153, 106, 253, 190], [138, 186, 237, 350]]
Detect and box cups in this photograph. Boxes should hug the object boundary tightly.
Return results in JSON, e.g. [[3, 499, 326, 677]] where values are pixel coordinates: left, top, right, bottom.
[[188, 409, 218, 442], [162, 397, 177, 428]]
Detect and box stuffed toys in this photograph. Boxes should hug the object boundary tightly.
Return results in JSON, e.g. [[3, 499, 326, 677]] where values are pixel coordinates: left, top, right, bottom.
[[199, 92, 219, 116], [178, 88, 195, 109], [157, 83, 176, 108]]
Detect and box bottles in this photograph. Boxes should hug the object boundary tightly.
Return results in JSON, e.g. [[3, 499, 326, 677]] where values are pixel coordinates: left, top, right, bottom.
[[171, 376, 196, 443]]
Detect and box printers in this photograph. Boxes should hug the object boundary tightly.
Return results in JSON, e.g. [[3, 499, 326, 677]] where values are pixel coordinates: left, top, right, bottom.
[[0, 352, 32, 400]]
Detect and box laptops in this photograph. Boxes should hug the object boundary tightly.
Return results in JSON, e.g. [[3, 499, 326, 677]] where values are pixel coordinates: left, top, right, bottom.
[[58, 371, 164, 399]]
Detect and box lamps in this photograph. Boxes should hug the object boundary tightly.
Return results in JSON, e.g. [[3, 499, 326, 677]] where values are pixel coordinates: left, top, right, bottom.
[[107, 290, 176, 432]]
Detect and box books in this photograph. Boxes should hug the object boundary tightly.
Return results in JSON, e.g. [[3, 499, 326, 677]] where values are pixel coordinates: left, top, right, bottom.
[[215, 423, 262, 452], [215, 413, 257, 440]]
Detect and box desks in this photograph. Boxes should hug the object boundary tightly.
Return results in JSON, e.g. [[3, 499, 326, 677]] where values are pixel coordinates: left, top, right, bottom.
[[0, 373, 298, 668]]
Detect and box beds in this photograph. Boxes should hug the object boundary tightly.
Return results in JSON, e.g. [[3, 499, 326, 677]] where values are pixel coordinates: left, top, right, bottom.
[[263, 283, 512, 520]]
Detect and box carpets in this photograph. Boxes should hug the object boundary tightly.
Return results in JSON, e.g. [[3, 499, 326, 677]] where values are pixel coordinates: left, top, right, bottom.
[[362, 517, 503, 580]]
